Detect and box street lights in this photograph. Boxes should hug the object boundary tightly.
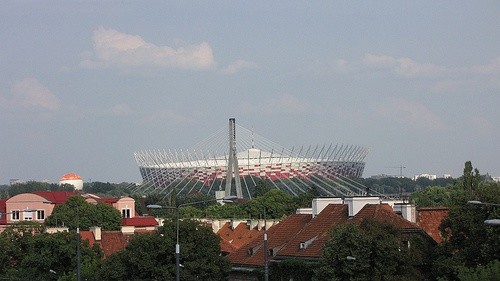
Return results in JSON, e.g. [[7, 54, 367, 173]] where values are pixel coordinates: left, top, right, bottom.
[[147, 204, 180, 281], [43, 201, 81, 281], [222, 197, 269, 281]]
